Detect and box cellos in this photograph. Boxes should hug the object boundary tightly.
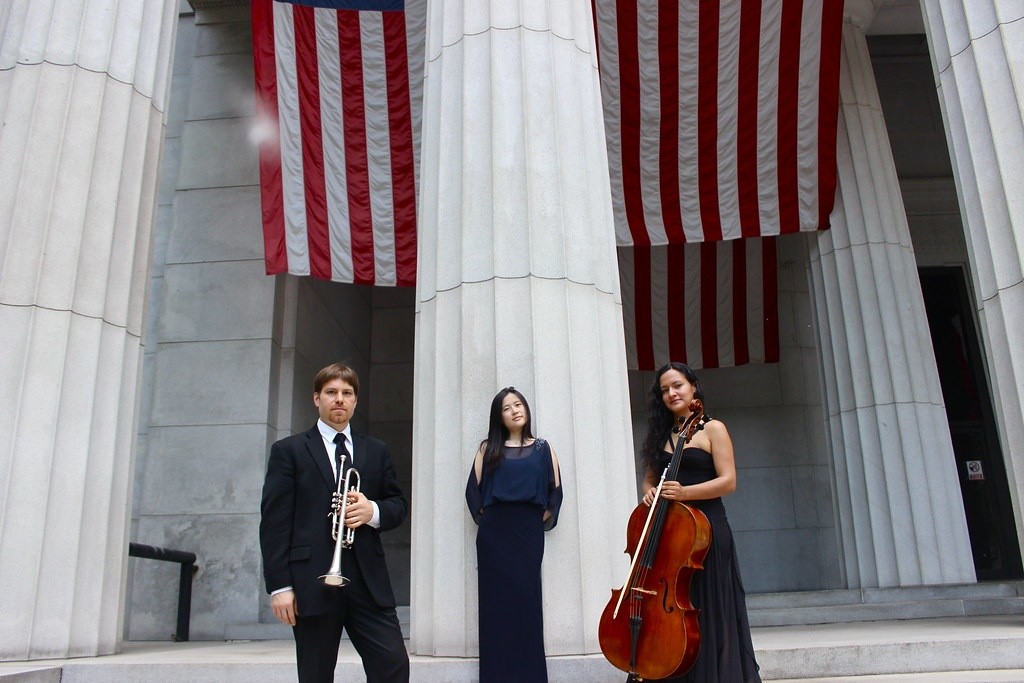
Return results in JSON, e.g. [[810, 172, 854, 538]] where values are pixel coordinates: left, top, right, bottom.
[[599, 398, 713, 682]]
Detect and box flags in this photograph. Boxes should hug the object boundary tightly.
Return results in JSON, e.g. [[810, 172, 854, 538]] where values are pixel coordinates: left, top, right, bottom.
[[616, 237, 779, 371], [252, 1, 428, 287], [592, 0, 843, 247]]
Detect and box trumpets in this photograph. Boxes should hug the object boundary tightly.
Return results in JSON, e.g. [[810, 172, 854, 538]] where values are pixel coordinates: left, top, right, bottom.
[[318, 454, 360, 587]]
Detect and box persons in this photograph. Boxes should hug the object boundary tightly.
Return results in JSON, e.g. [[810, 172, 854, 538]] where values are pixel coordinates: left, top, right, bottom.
[[259, 364, 410, 682], [464, 386, 564, 682], [626, 363, 764, 683]]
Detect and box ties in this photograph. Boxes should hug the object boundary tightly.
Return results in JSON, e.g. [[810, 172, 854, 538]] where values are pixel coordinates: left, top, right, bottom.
[[333, 433, 354, 498]]
